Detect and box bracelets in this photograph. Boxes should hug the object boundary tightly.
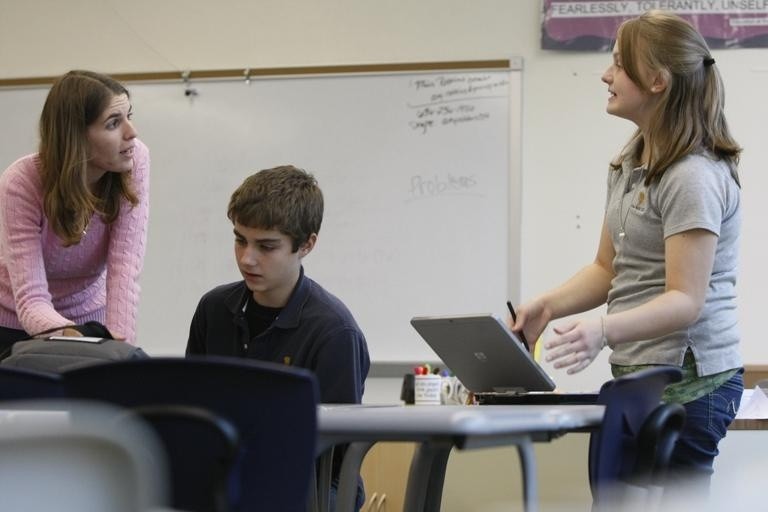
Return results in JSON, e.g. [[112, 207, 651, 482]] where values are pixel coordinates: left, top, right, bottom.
[[600, 316, 608, 350]]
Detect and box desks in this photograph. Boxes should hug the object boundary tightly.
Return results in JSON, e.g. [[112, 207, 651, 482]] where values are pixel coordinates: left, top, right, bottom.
[[306, 396, 609, 511]]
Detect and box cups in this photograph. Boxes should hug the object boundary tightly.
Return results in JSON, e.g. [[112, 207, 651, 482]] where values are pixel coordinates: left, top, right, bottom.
[[414, 374, 469, 404]]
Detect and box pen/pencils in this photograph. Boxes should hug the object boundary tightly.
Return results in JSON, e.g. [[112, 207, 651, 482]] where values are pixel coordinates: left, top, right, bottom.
[[507, 300, 530, 353], [415, 364, 448, 377]]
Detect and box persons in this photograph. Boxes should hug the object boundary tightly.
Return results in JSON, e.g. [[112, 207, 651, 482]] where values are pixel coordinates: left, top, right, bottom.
[[1, 68, 151, 361], [506, 7, 745, 512], [185, 165, 371, 512]]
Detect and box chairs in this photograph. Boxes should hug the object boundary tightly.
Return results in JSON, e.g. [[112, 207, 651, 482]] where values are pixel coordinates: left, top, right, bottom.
[[61, 354, 324, 512]]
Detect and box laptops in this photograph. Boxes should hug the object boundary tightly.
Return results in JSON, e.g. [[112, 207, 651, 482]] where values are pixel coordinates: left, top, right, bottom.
[[410, 312, 600, 405]]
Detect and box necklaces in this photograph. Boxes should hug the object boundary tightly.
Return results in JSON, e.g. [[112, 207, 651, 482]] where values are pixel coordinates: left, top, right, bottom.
[[617, 165, 643, 241]]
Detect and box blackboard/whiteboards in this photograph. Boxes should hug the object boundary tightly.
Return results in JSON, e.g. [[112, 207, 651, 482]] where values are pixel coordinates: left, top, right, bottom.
[[0, 59, 510, 378], [521, 0, 767, 388]]
